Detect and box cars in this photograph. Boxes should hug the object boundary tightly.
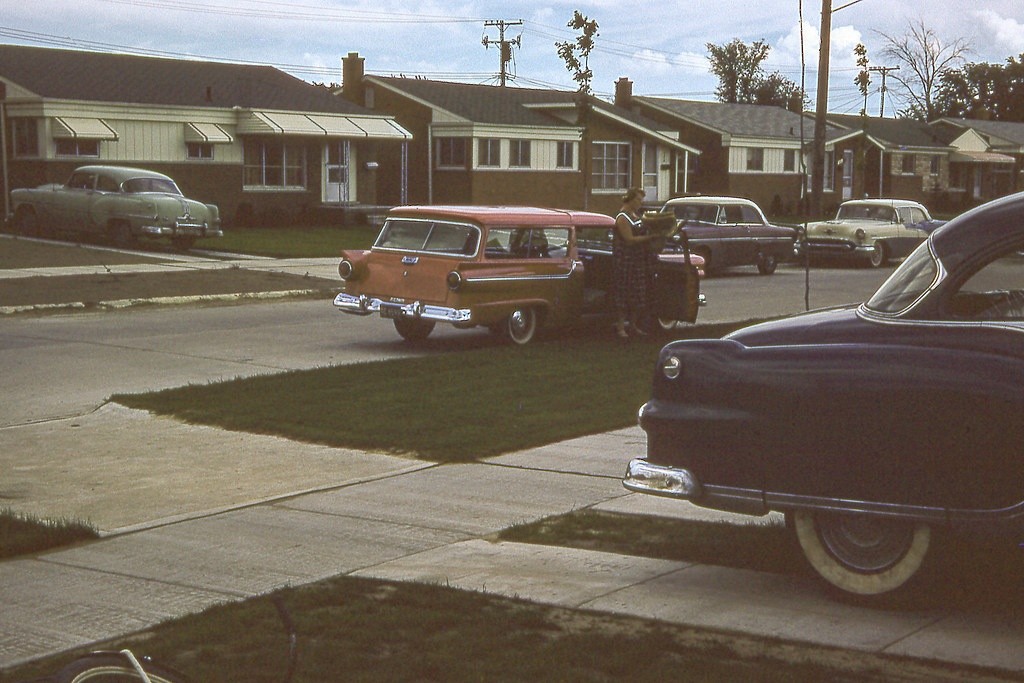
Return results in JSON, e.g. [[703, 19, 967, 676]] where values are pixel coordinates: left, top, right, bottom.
[[334, 202, 707, 349], [794, 200, 952, 271], [621, 191, 1022, 603], [654, 196, 796, 275], [8, 165, 225, 250]]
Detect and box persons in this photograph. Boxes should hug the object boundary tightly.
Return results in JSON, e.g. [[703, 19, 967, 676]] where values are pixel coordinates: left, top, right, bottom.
[[612, 187, 667, 338]]
